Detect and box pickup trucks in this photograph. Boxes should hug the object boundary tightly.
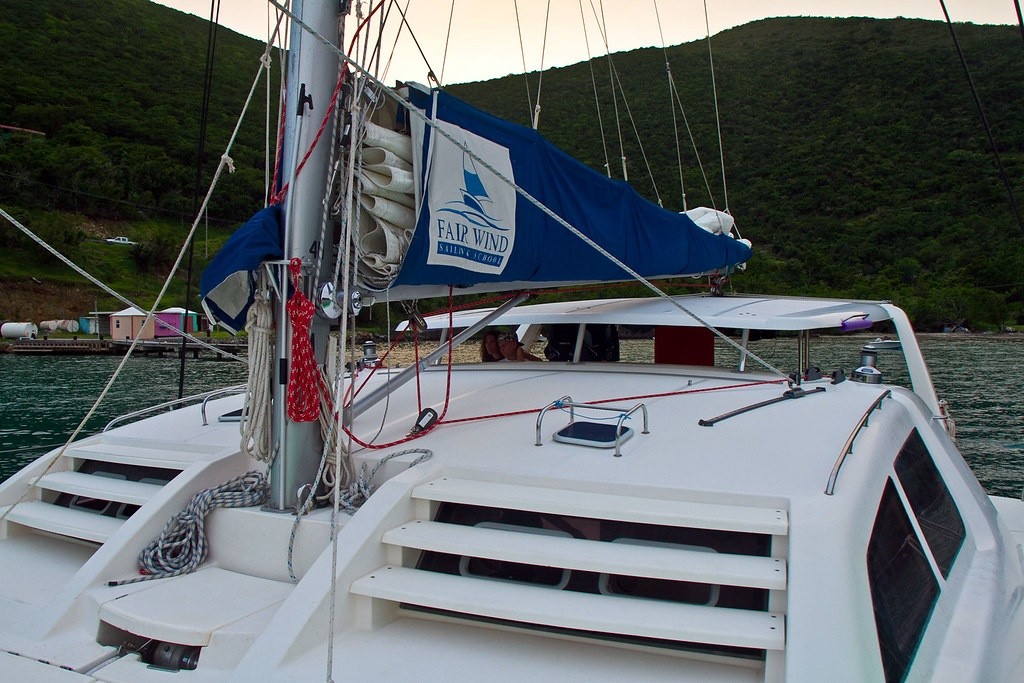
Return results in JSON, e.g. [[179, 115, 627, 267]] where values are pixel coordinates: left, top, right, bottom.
[[105, 235, 138, 245]]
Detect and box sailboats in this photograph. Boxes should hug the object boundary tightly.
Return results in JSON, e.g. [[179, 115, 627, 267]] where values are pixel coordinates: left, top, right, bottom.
[[0, 0, 1024, 681]]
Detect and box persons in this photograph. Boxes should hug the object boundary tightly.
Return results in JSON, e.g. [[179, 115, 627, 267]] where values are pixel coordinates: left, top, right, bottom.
[[479, 329, 542, 362], [30, 329, 37, 339], [495, 330, 532, 362]]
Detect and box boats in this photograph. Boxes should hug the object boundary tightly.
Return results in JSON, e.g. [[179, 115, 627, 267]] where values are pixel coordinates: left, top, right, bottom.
[[866, 338, 905, 351]]
